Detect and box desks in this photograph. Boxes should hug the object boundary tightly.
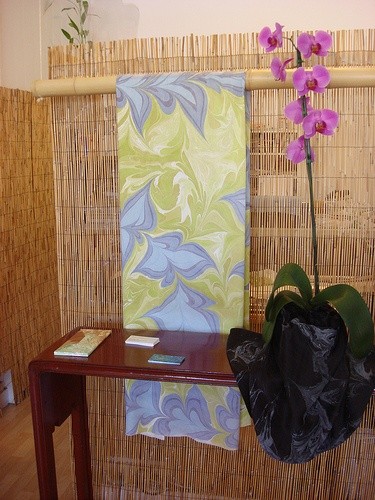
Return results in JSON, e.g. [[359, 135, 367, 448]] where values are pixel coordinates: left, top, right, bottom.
[[28, 326, 254, 500]]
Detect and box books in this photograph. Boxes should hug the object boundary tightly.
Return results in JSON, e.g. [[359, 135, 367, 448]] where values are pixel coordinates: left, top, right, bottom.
[[54, 329, 112, 358]]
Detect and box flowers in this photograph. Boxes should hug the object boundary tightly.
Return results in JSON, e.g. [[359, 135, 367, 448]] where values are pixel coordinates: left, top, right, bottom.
[[256, 22, 375, 357]]
